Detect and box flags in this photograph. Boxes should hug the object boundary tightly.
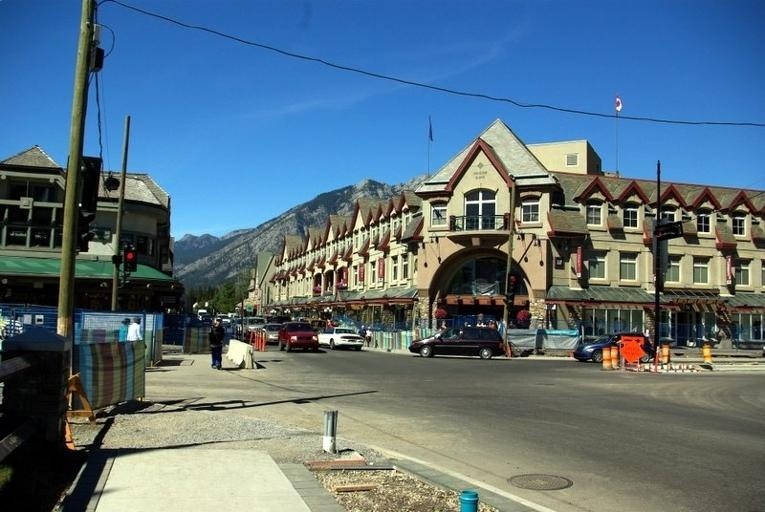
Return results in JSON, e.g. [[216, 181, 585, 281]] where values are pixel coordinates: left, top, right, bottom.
[[428, 116, 434, 142], [616, 95, 624, 112]]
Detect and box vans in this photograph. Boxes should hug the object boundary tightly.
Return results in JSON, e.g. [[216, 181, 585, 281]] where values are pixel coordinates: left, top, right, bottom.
[[407, 325, 506, 361]]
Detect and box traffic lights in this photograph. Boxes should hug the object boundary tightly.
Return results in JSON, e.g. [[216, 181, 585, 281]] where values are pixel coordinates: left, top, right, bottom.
[[123, 247, 139, 273], [503, 293, 515, 312], [76, 211, 96, 253], [507, 272, 519, 292]]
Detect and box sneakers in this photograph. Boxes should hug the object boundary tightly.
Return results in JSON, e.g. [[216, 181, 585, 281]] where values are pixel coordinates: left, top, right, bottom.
[[124, 316, 142, 322], [212, 364, 222, 370]]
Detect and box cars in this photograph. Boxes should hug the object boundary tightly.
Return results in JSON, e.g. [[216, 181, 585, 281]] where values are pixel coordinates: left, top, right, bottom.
[[574, 331, 656, 366], [183, 305, 366, 356]]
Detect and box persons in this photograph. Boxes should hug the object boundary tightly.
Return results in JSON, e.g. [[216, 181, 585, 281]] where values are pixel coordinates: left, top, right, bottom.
[[118, 316, 130, 338], [439, 319, 502, 331], [128, 316, 143, 344], [365, 330, 374, 348], [207, 315, 226, 368]]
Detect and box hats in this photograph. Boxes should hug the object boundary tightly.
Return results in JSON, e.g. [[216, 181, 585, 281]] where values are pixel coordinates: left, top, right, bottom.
[[216, 317, 223, 321]]
